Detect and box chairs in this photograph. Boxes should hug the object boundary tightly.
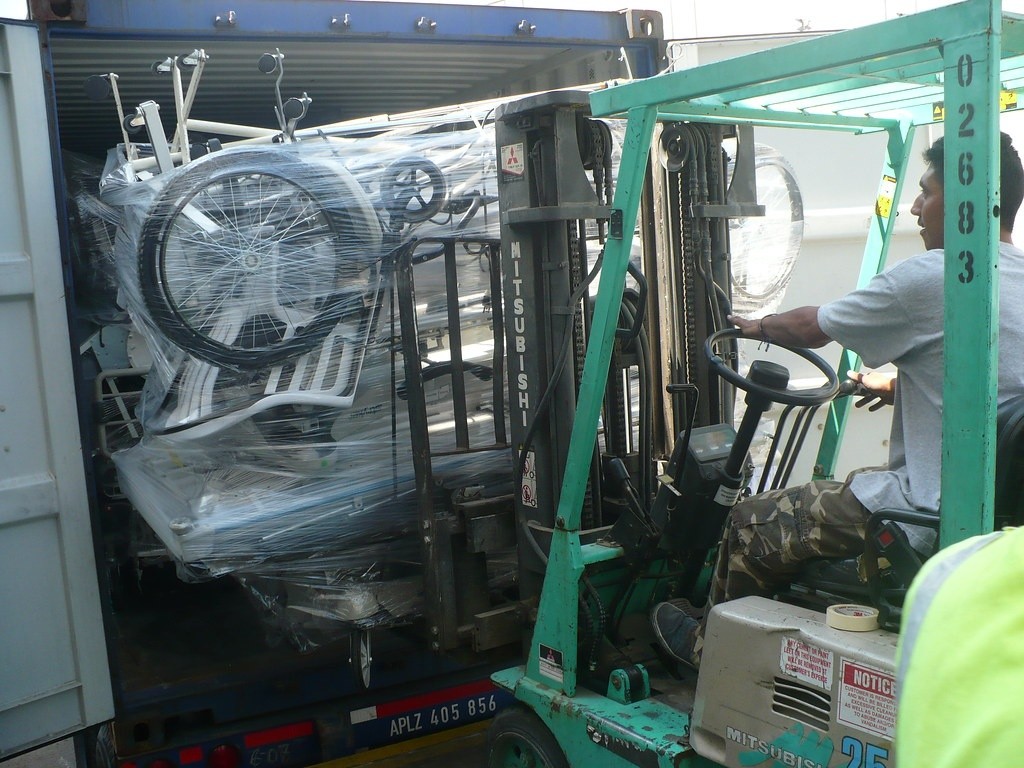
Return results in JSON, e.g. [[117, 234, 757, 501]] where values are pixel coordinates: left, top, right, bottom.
[[764, 394, 1024, 614]]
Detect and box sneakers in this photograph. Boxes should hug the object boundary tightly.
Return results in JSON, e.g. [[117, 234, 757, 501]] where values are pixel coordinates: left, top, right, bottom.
[[652, 600, 704, 670]]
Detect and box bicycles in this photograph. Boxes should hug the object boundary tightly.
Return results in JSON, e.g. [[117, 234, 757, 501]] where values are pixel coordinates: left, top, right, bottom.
[[136, 116, 804, 401]]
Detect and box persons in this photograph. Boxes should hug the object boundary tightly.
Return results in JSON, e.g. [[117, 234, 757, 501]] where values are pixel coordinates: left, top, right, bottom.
[[649, 132, 1024, 671]]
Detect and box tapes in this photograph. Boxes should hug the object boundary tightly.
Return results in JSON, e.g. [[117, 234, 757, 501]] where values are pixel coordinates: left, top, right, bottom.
[[825, 604, 882, 633]]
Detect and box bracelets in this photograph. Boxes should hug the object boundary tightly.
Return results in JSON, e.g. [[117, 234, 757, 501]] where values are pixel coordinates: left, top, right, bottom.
[[757, 313, 781, 353]]
